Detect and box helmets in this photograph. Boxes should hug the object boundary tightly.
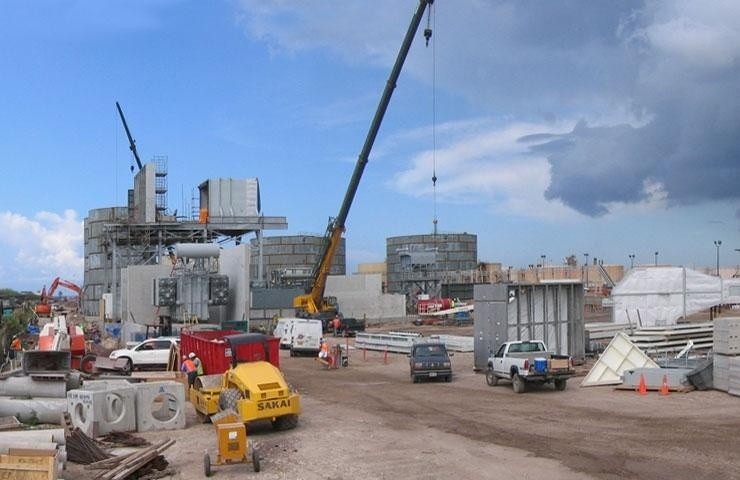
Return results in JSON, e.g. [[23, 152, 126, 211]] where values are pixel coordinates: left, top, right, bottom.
[[183, 352, 195, 360]]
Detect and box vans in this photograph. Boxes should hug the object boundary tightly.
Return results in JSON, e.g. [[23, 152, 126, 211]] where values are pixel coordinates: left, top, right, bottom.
[[272, 317, 296, 350], [289, 318, 324, 358]]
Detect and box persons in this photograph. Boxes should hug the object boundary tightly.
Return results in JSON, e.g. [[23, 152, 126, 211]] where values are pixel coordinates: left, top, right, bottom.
[[333, 314, 341, 337], [91, 330, 101, 353], [180, 355, 198, 388], [189, 352, 204, 376], [319, 338, 331, 370], [10, 335, 22, 367], [258, 323, 266, 333]]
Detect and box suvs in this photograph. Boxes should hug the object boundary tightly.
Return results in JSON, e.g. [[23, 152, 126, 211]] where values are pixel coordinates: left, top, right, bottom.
[[108, 335, 181, 373]]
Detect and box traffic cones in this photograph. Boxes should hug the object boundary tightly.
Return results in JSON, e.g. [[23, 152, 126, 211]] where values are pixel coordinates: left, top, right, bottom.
[[659, 374, 670, 396], [637, 373, 648, 395]]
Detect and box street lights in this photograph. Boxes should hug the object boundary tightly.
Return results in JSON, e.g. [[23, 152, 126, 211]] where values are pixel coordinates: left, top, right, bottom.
[[526, 240, 722, 276]]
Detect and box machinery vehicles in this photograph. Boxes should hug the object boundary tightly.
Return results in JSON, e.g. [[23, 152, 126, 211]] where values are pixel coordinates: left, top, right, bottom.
[[33, 275, 81, 317], [291, 0, 437, 333], [187, 332, 303, 432]]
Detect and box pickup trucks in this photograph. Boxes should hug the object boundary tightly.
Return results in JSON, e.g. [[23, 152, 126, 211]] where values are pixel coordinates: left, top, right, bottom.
[[406, 340, 455, 385], [485, 339, 577, 394]]
